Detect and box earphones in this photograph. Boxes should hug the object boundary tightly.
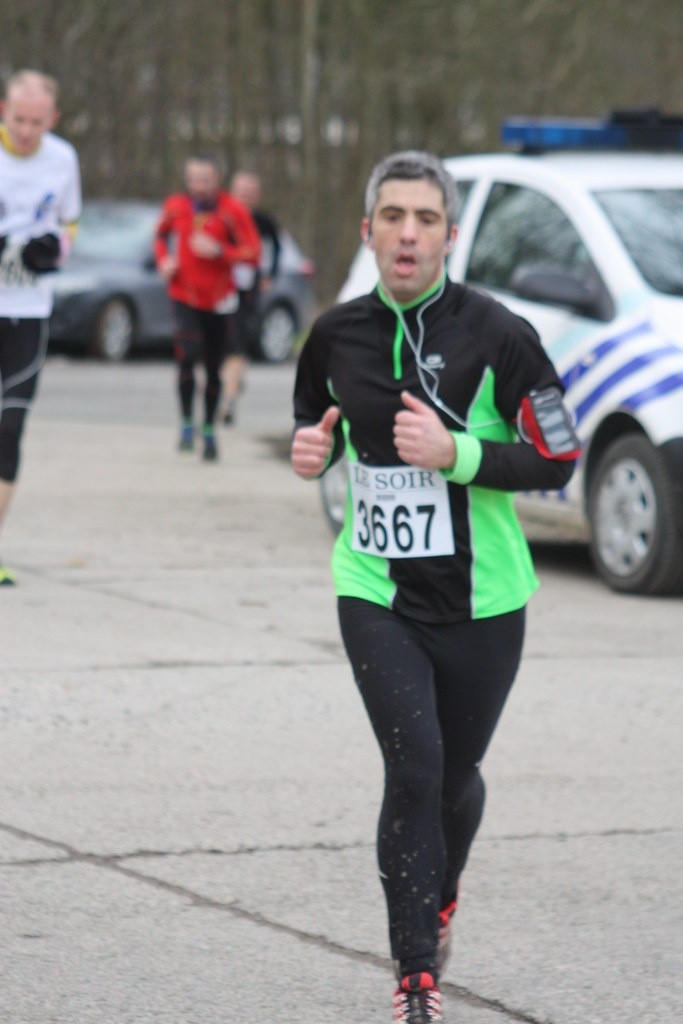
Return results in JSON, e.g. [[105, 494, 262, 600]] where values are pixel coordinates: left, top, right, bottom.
[[364, 233, 373, 244], [445, 238, 453, 250]]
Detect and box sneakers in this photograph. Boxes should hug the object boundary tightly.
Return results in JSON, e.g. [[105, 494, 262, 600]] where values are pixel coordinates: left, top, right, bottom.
[[392, 971, 442, 1024], [435, 900, 456, 971]]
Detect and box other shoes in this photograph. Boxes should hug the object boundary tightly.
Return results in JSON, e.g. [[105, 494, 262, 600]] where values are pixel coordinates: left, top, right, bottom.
[[200, 425, 216, 461], [178, 423, 196, 452], [0, 565, 14, 584]]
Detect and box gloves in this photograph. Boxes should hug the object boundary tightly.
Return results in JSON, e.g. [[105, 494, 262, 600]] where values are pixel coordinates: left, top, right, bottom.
[[22, 234, 60, 273]]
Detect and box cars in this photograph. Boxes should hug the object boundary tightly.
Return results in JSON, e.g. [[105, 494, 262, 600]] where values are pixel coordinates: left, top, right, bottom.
[[49, 197, 320, 364], [317, 116, 683, 606]]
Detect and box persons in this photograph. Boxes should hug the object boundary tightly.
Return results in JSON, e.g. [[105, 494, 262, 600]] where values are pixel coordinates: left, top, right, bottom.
[[287, 152, 589, 1024], [147, 155, 285, 467], [0, 69, 83, 585]]
